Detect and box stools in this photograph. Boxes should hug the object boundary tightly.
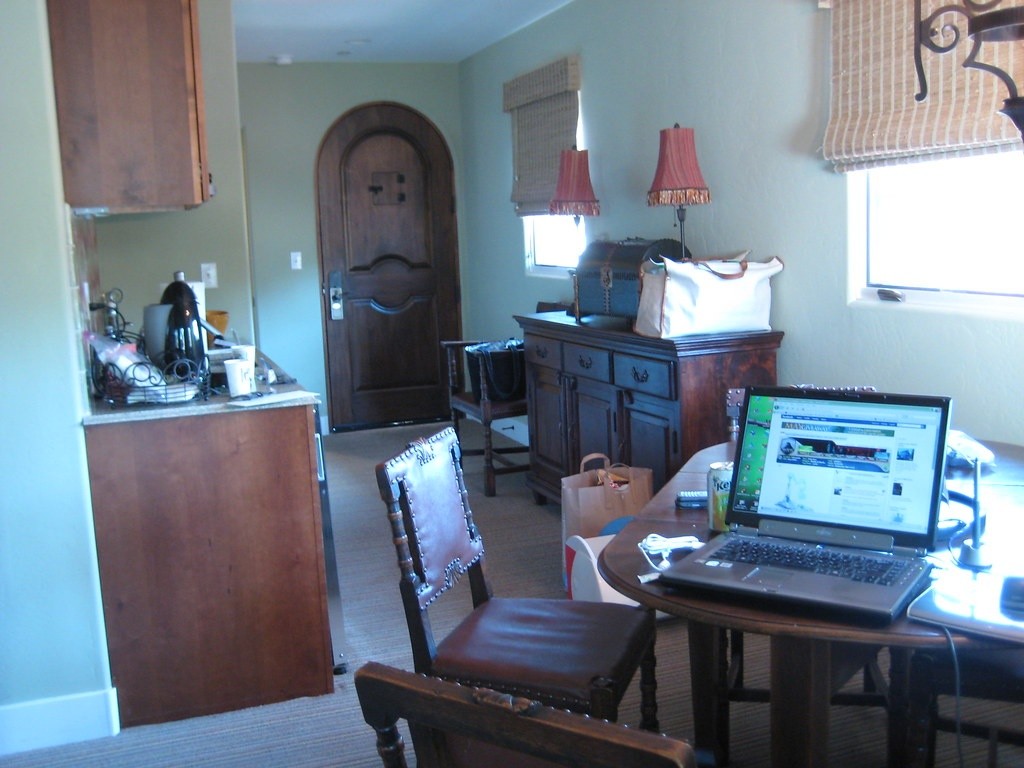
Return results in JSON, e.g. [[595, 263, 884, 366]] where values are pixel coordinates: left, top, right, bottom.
[[909, 643, 1024, 768]]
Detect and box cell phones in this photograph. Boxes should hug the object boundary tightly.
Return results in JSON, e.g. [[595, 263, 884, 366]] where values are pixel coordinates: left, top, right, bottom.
[[676, 491, 707, 501]]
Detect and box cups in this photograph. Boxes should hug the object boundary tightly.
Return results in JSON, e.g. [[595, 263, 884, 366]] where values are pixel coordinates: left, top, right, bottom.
[[223, 360, 249, 398], [206, 310, 228, 348], [231, 345, 256, 393]]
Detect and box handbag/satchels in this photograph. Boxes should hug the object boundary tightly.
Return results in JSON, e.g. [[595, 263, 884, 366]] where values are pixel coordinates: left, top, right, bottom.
[[634, 249, 784, 338], [464, 338, 525, 404], [561, 453, 654, 590]]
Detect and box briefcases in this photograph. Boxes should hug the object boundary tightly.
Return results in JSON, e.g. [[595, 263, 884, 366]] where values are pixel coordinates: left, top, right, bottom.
[[574, 237, 692, 318]]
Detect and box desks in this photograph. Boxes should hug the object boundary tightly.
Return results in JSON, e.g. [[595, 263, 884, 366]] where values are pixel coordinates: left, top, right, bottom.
[[595, 440, 1018, 768]]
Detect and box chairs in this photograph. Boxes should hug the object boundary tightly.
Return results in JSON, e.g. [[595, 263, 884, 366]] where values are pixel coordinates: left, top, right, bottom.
[[354, 663, 698, 768], [375, 427, 659, 732], [440, 335, 529, 497]]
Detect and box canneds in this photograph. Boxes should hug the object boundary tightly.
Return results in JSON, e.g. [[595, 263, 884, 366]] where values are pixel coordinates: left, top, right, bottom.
[[707, 462, 734, 532]]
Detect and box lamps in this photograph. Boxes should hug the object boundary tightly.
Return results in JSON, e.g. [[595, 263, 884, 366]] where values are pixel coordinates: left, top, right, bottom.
[[550, 146, 602, 268], [644, 120, 712, 265]]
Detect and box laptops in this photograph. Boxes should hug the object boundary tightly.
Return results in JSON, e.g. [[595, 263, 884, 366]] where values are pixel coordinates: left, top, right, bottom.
[[661, 385, 953, 623]]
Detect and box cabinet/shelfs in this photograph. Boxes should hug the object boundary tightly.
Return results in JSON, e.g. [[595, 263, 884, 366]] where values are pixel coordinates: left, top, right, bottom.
[[45, 1, 209, 211], [513, 313, 784, 514]]
